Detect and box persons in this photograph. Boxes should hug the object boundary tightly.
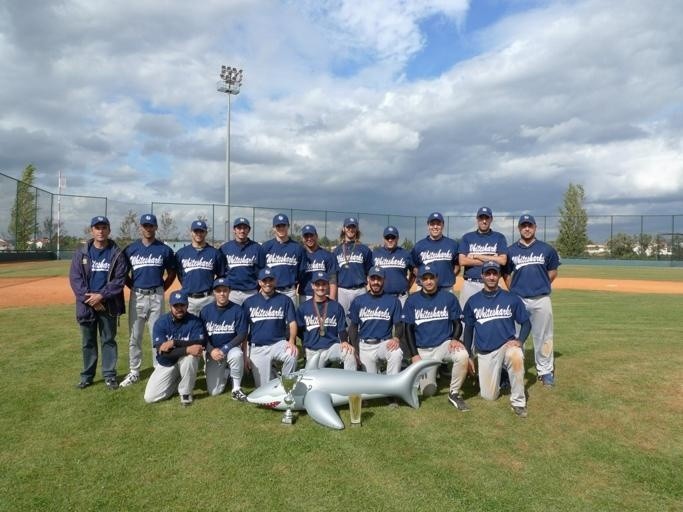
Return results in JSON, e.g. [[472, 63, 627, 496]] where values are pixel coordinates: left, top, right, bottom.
[[117, 214, 174, 388], [260, 212, 306, 310], [332, 216, 372, 310], [504, 214, 560, 386], [410, 212, 460, 299], [217, 217, 262, 304], [299, 224, 336, 303], [199, 277, 247, 402], [142, 288, 202, 404], [67, 214, 129, 390], [348, 267, 404, 376], [174, 220, 227, 314], [295, 272, 358, 373], [238, 268, 298, 389], [372, 227, 412, 293], [461, 260, 532, 417], [457, 207, 510, 351], [400, 265, 468, 413]]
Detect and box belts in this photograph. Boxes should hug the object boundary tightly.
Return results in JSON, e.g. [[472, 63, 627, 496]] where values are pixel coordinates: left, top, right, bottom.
[[188, 291, 212, 298], [133, 286, 157, 295], [346, 283, 365, 289], [394, 292, 407, 297], [465, 278, 484, 282], [275, 286, 292, 291]]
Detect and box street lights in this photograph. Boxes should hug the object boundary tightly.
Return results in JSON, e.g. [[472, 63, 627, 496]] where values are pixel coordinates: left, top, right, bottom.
[[216, 64, 244, 241]]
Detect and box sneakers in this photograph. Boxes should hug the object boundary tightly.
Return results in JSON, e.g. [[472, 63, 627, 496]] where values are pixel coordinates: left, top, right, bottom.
[[231, 388, 248, 402], [119, 371, 140, 388], [77, 380, 93, 389], [510, 405, 528, 418], [180, 394, 193, 404], [271, 362, 281, 378], [104, 376, 118, 389], [448, 390, 470, 412], [541, 373, 555, 387]]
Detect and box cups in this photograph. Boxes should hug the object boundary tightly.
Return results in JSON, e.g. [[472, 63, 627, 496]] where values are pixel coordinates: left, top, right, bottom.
[[348, 392, 361, 424]]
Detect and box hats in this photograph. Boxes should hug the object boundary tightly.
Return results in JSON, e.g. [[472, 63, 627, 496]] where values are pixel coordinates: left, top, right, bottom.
[[258, 267, 275, 281], [233, 214, 317, 237], [476, 206, 536, 274], [417, 264, 437, 279], [212, 277, 231, 289], [90, 215, 110, 228], [427, 212, 444, 224], [311, 271, 330, 283], [191, 220, 207, 232], [140, 214, 158, 227], [169, 290, 189, 306]]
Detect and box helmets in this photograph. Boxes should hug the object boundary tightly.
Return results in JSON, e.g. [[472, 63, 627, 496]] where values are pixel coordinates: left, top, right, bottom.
[[343, 217, 398, 279]]
